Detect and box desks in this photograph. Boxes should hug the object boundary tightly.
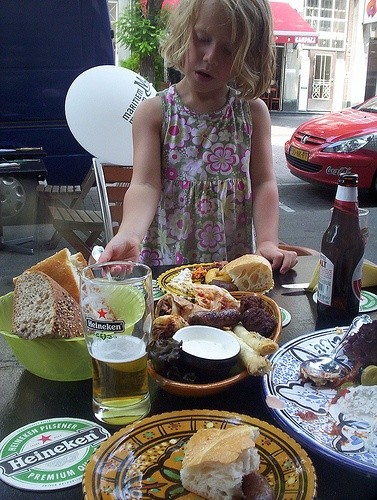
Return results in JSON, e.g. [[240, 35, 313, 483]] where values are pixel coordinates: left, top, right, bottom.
[[0, 159, 47, 256]]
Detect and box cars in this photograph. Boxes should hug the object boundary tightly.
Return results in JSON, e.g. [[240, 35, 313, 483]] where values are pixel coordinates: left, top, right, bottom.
[[285, 94, 377, 201]]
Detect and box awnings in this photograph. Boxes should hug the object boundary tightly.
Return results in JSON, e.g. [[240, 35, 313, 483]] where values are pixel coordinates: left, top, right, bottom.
[[139, 0, 318, 44]]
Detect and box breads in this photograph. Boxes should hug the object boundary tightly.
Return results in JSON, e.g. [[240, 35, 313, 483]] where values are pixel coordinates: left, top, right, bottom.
[[180, 424, 260, 500], [241, 295, 278, 336], [186, 284, 240, 310], [225, 254, 273, 292], [12, 248, 117, 339]]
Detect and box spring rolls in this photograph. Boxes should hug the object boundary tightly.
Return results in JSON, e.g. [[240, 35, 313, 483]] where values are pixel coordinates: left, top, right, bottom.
[[150, 293, 211, 339]]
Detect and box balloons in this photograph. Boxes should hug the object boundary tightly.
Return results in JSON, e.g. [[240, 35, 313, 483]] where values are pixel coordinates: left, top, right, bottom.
[[65, 65, 159, 165]]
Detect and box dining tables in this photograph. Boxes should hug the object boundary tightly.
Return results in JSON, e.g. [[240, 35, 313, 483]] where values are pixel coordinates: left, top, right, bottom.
[[0, 253, 377, 500]]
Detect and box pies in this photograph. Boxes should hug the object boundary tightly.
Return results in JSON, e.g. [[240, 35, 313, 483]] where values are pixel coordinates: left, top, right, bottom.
[[221, 325, 278, 375]]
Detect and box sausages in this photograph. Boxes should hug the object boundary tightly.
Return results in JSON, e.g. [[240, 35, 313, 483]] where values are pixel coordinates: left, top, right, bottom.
[[208, 279, 238, 292], [187, 308, 241, 327], [242, 472, 274, 500]]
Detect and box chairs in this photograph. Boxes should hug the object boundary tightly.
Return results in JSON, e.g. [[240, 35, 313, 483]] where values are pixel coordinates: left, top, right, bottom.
[[37, 164, 104, 261], [259, 80, 281, 111], [92, 158, 132, 246]]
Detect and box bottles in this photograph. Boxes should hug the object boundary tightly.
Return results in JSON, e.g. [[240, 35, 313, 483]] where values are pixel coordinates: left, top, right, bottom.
[[316, 173, 365, 323]]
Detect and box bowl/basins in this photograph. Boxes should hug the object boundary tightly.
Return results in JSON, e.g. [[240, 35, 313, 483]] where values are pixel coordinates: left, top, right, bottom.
[[0, 279, 146, 381], [172, 325, 241, 376], [148, 291, 282, 397]]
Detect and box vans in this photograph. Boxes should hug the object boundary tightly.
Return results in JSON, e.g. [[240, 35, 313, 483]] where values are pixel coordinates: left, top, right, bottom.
[[1, 1, 115, 225]]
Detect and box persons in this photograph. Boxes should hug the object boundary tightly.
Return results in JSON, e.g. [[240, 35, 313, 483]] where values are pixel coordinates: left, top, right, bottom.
[[99, 0, 299, 279]]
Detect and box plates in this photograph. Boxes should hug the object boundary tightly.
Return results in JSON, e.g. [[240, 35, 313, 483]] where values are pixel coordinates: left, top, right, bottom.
[[82, 409, 319, 500], [261, 326, 377, 480], [156, 262, 274, 298]]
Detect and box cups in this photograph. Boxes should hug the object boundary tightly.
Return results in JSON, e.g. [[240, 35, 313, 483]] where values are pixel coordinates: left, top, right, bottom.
[[331, 207, 369, 251], [78, 260, 151, 425]]
